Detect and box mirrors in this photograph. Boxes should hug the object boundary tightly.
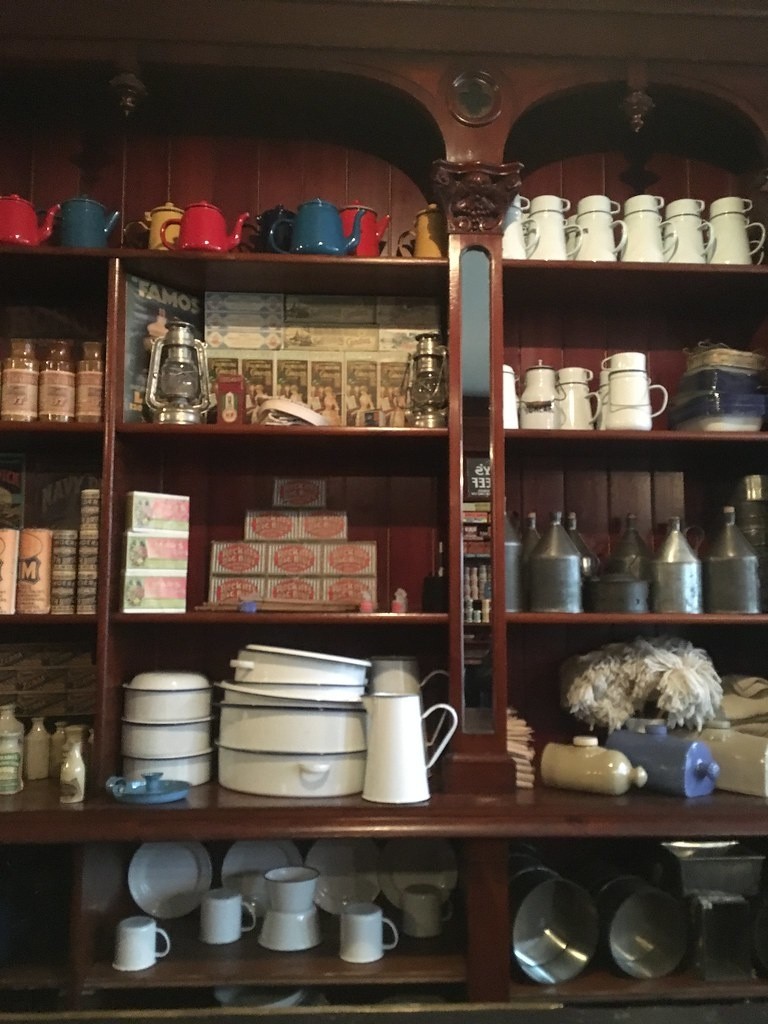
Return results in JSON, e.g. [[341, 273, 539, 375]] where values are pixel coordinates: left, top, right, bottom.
[[460, 250, 493, 733]]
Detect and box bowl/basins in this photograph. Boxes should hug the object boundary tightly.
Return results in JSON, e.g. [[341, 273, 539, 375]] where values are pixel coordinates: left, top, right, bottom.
[[215, 643, 371, 798], [120, 670, 215, 788]]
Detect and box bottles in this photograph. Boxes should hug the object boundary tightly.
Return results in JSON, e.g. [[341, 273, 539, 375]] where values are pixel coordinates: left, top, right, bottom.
[[521, 360, 560, 429], [2, 335, 104, 425], [0, 705, 95, 804]]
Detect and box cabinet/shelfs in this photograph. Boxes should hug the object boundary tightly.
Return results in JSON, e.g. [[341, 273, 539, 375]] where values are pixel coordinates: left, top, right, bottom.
[[1, 35, 768, 1024]]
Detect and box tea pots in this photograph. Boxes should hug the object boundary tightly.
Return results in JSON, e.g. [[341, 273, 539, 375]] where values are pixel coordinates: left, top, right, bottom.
[[338, 198, 391, 257], [160, 199, 250, 251], [0, 194, 62, 247], [124, 201, 184, 251], [396, 203, 446, 258], [36, 192, 120, 247], [237, 204, 297, 254], [270, 197, 366, 256]]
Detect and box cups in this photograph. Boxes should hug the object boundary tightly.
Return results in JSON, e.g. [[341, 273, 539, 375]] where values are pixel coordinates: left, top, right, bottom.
[[257, 910, 322, 951], [663, 199, 716, 264], [599, 351, 669, 430], [399, 883, 455, 938], [502, 193, 541, 261], [577, 196, 627, 262], [111, 916, 170, 970], [501, 365, 521, 429], [339, 901, 398, 963], [529, 195, 584, 261], [197, 888, 255, 945], [623, 195, 678, 262], [709, 197, 766, 265], [555, 367, 603, 431], [265, 866, 321, 911]]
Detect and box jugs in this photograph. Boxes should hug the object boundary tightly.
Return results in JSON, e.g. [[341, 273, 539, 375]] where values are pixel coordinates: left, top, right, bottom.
[[367, 655, 452, 779], [360, 692, 459, 803]]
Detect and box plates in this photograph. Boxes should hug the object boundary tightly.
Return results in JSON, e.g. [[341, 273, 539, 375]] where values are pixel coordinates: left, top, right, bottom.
[[126, 842, 213, 918], [380, 838, 458, 911], [213, 988, 306, 1009], [219, 840, 303, 916], [304, 838, 381, 915]]
